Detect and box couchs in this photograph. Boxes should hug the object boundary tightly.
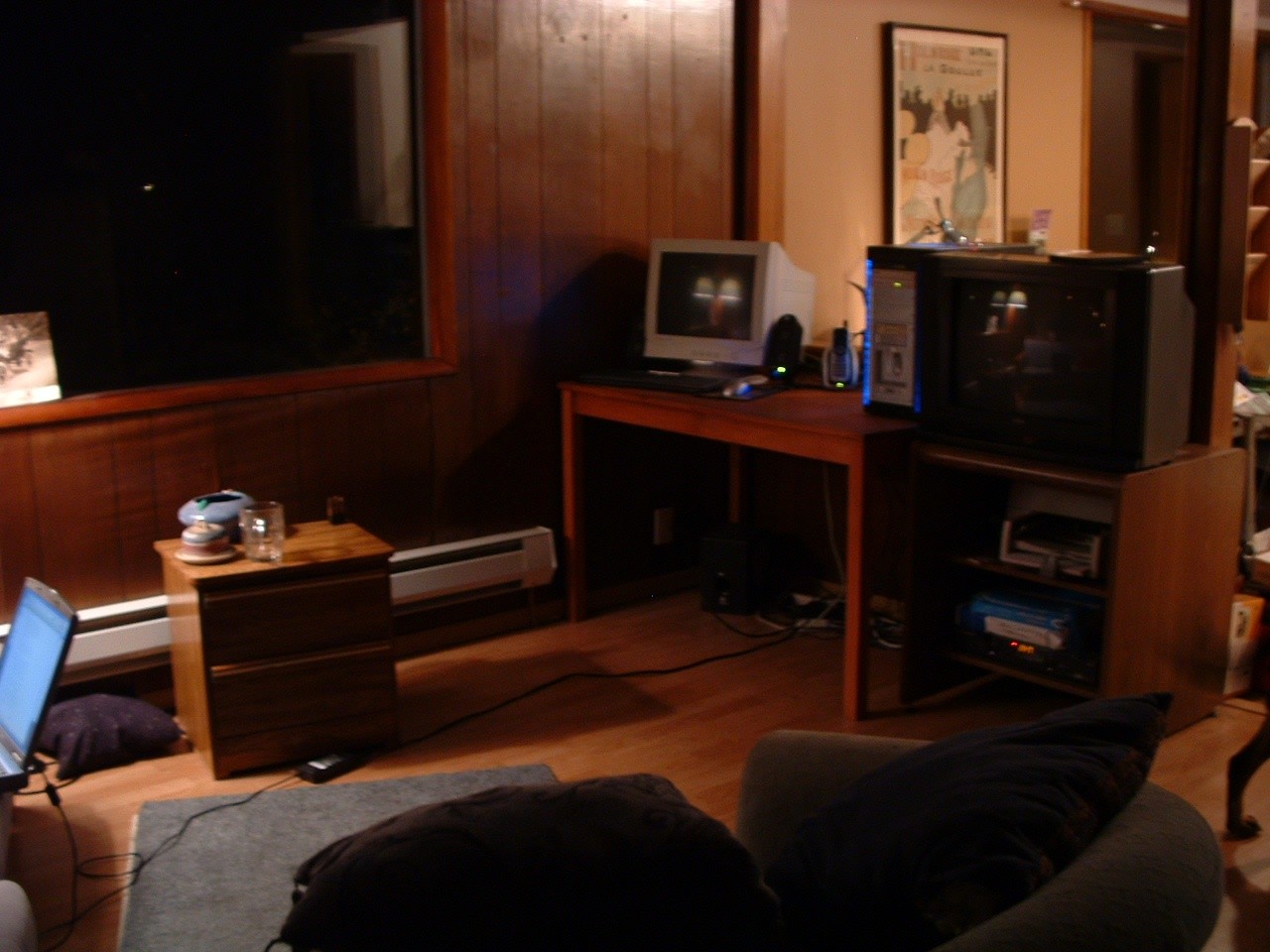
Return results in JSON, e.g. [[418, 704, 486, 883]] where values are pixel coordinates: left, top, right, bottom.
[[737, 728, 1222, 952]]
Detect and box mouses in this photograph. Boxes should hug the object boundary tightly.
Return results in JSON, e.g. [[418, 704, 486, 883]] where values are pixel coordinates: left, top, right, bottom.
[[720, 379, 754, 397]]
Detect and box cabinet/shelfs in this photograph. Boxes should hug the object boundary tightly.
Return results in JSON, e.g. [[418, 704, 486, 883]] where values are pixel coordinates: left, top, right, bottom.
[[901, 434, 1246, 736], [152, 519, 395, 780]]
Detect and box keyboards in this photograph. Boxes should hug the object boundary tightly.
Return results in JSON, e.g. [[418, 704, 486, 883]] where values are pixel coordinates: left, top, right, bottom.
[[576, 369, 726, 394]]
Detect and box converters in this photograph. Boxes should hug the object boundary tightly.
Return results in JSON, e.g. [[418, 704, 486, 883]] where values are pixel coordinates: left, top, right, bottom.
[[299, 751, 357, 784]]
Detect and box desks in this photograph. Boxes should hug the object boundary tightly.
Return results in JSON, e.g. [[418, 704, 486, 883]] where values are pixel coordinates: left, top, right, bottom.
[[559, 372, 915, 722]]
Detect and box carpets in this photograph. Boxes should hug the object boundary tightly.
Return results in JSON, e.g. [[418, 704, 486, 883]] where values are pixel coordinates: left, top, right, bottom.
[[119, 762, 559, 952]]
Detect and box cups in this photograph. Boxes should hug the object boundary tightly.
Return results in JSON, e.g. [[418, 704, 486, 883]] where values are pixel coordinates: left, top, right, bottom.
[[239, 500, 285, 562]]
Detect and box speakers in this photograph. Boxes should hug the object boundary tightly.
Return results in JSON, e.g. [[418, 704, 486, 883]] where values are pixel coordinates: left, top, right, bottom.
[[767, 314, 803, 387]]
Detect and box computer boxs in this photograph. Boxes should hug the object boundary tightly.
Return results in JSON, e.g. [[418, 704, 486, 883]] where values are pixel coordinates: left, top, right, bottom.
[[863, 244, 975, 419]]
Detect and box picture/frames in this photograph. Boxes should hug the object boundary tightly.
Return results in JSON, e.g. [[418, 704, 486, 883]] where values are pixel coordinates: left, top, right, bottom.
[[882, 20, 1007, 243]]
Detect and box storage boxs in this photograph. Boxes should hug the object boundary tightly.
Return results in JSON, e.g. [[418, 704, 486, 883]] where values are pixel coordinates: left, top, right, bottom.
[[1226, 589, 1265, 698]]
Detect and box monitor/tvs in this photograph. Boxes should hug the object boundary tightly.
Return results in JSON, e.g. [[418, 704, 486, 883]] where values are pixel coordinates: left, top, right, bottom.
[[641, 238, 815, 382], [928, 252, 1196, 469]]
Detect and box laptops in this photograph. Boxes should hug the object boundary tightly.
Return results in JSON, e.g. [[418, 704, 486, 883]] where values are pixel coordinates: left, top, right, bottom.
[[0, 576, 80, 792]]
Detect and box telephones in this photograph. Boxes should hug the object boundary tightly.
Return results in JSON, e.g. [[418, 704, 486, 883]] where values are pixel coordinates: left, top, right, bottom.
[[823, 326, 860, 391]]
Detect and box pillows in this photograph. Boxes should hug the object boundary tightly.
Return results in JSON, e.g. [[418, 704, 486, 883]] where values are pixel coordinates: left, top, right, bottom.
[[277, 693, 1173, 952], [36, 692, 184, 779]]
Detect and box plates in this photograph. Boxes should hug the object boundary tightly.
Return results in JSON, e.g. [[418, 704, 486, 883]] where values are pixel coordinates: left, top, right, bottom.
[[174, 545, 238, 563]]
